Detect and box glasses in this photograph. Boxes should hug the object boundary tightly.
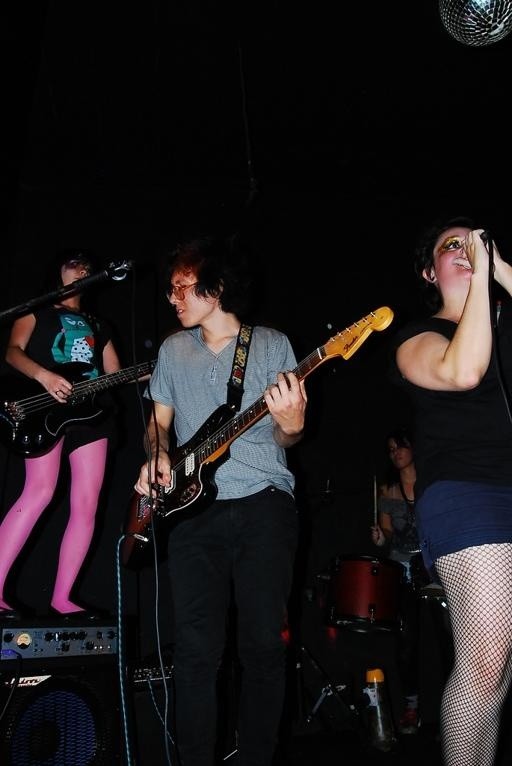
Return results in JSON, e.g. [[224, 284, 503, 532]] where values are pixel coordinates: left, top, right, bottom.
[[166, 281, 198, 301]]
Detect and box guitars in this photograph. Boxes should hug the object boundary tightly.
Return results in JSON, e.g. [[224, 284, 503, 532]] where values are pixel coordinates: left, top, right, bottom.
[[123, 307, 394, 567], [0, 361, 159, 459]]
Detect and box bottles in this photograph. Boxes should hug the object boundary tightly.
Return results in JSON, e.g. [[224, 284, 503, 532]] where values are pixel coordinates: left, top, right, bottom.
[[363, 668, 392, 752]]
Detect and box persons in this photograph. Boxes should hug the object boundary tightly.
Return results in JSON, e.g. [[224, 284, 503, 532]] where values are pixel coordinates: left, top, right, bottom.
[[392, 220, 509, 764], [372, 428, 420, 553], [133, 250, 305, 765], [0, 257, 150, 615]]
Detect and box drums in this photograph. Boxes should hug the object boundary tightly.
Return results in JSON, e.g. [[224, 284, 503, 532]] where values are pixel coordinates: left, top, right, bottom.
[[335, 558, 408, 633]]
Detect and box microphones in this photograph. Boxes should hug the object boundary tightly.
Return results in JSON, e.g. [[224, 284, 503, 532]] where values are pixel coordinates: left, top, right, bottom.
[[108, 255, 136, 281]]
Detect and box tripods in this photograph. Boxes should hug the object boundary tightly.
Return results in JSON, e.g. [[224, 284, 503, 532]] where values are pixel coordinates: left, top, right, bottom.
[[288, 658, 431, 750]]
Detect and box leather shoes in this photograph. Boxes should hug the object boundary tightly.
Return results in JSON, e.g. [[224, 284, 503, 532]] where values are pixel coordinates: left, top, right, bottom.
[[1, 597, 23, 625], [44, 602, 103, 627]]
